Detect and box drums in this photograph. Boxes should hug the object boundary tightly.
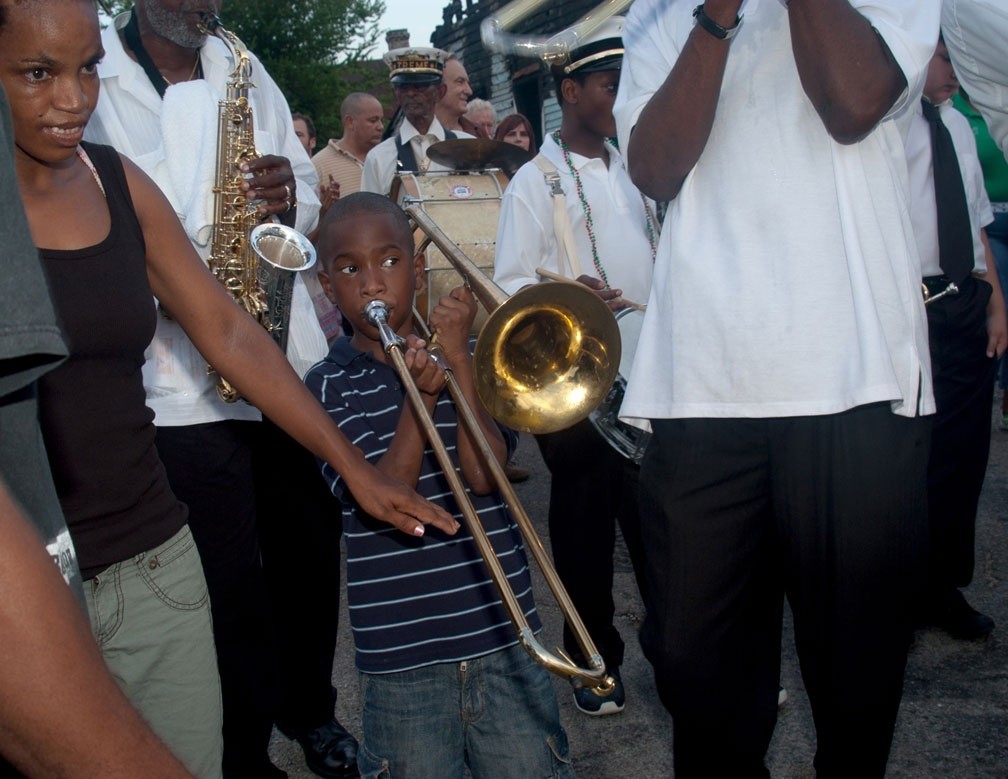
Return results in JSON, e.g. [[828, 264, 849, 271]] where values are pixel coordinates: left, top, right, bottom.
[[387, 166, 516, 340]]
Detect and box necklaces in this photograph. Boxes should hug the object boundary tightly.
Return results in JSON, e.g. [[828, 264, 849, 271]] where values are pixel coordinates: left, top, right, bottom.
[[553, 125, 659, 299]]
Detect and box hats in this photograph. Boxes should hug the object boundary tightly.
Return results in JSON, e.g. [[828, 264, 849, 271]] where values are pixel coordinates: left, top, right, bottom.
[[542, 16, 629, 76], [384, 47, 447, 86]]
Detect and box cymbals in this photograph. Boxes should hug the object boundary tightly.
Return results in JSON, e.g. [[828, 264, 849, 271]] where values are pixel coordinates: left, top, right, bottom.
[[424, 135, 531, 175]]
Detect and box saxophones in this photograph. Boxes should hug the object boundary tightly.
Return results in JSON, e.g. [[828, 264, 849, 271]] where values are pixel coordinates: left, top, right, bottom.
[[193, 9, 320, 405]]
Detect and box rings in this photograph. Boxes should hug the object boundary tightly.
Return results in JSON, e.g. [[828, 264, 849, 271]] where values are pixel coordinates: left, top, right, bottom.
[[283, 185, 292, 200], [283, 201, 292, 214]]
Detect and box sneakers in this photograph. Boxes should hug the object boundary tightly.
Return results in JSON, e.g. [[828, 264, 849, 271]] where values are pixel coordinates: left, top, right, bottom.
[[570, 664, 627, 714]]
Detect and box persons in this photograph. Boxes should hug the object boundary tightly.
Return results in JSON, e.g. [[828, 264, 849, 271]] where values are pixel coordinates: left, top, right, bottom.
[[895, 27, 1007, 648], [299, 192, 583, 779], [82, 0, 362, 779], [936, 0, 1008, 162], [2, 1, 468, 779], [495, 30, 672, 719], [432, 51, 482, 138], [605, 0, 944, 779], [312, 92, 386, 197], [493, 113, 536, 158], [297, 176, 346, 352], [363, 45, 479, 197], [954, 84, 1007, 434], [0, 95, 209, 779], [463, 97, 496, 140], [290, 113, 319, 158]]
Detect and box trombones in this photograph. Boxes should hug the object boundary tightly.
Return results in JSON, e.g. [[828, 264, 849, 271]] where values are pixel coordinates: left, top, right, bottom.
[[359, 205, 625, 699]]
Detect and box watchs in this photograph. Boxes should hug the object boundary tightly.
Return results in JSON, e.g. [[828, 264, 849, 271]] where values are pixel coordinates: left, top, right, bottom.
[[693, 3, 746, 40]]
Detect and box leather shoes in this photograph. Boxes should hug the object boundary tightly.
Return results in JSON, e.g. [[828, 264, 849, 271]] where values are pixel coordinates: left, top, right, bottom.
[[304, 718, 361, 777], [922, 591, 993, 643]]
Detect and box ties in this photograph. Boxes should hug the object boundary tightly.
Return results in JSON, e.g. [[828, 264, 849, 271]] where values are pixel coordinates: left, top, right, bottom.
[[921, 100, 976, 290]]
